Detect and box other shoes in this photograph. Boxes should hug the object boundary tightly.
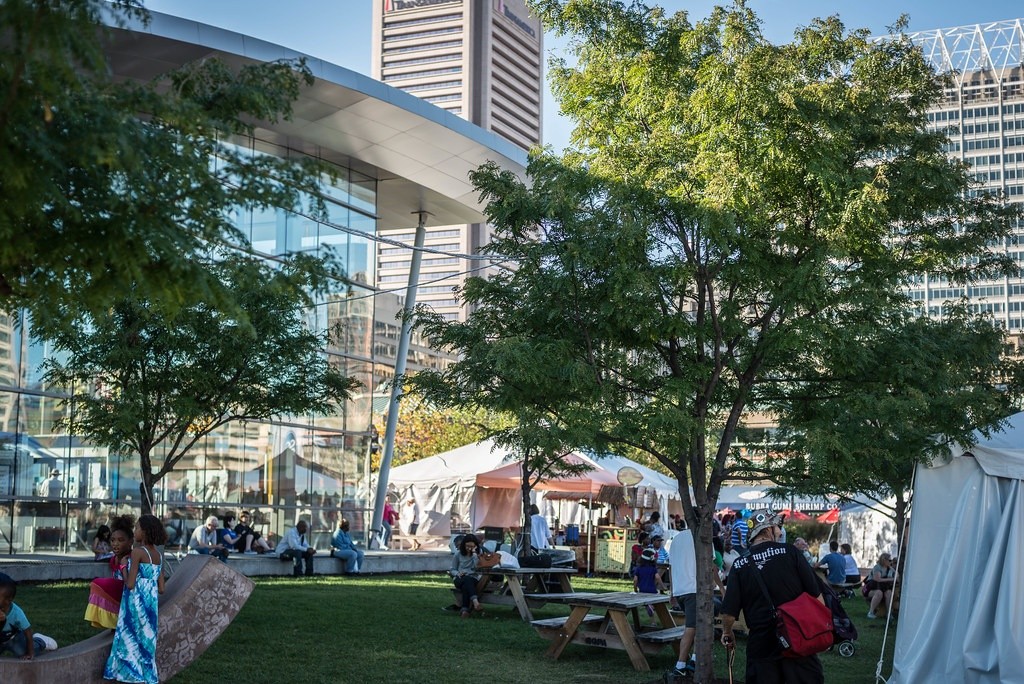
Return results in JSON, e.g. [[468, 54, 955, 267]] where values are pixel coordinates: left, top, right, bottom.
[[462, 611, 470, 618], [837, 589, 853, 599], [379, 544, 391, 551], [264, 549, 276, 555], [408, 542, 421, 552], [473, 604, 482, 611], [867, 612, 878, 620], [441, 604, 462, 612], [244, 549, 257, 555]]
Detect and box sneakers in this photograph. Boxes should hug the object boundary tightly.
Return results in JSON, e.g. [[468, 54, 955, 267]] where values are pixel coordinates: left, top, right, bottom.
[[645, 604, 654, 617], [32, 633, 58, 651], [673, 653, 696, 676]]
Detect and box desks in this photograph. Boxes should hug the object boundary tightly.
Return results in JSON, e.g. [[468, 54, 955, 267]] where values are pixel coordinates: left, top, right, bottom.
[[714, 585, 750, 635], [469, 567, 575, 622], [547, 592, 692, 672], [812, 565, 828, 585], [550, 545, 586, 567]]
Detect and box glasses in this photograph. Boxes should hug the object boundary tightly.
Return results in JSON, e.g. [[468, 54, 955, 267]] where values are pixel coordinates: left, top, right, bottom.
[[886, 559, 893, 563], [657, 539, 664, 542]]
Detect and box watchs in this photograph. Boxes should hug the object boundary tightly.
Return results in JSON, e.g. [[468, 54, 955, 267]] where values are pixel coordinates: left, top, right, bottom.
[[120, 564, 127, 572]]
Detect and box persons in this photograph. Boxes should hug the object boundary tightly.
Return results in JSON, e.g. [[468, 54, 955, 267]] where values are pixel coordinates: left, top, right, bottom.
[[407, 498, 421, 551], [668, 506, 726, 677], [0, 572, 57, 660], [629, 511, 910, 627], [40, 468, 62, 502], [720, 514, 826, 684], [379, 495, 395, 550], [188, 516, 229, 564], [275, 520, 316, 578], [530, 504, 555, 554], [443, 534, 482, 617], [330, 519, 364, 577], [221, 511, 275, 555], [84, 514, 165, 684]]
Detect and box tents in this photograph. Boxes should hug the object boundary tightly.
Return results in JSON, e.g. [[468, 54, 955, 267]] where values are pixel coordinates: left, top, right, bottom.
[[875, 411, 1024, 684], [355, 419, 913, 573]]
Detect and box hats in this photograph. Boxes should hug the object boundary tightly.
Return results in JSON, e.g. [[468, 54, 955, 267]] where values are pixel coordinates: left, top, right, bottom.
[[50, 468, 62, 475], [651, 534, 661, 544], [638, 532, 648, 544], [747, 508, 787, 540]]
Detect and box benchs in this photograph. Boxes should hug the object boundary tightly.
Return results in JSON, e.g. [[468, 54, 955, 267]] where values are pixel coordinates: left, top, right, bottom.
[[530, 614, 605, 630], [524, 593, 597, 600], [450, 586, 526, 592], [832, 583, 861, 586], [669, 609, 684, 613], [637, 625, 686, 642], [391, 535, 450, 549], [0, 551, 454, 581]]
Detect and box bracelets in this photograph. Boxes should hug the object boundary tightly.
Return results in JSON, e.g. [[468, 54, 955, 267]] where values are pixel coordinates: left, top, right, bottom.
[[472, 551, 477, 554]]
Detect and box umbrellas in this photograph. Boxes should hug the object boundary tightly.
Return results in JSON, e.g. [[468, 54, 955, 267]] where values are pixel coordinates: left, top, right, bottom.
[[776, 508, 811, 522], [716, 507, 736, 516], [818, 508, 839, 523]]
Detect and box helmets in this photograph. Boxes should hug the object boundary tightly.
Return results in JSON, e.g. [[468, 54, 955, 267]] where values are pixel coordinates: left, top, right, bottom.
[[642, 549, 658, 565]]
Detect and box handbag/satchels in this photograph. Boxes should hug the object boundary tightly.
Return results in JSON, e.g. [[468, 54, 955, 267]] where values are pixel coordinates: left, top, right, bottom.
[[479, 552, 502, 568], [517, 553, 552, 569], [862, 584, 870, 598], [493, 550, 521, 569], [777, 591, 835, 657]]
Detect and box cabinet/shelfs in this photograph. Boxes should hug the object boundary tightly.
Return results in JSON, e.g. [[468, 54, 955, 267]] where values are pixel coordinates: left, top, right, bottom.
[[577, 534, 596, 553]]
